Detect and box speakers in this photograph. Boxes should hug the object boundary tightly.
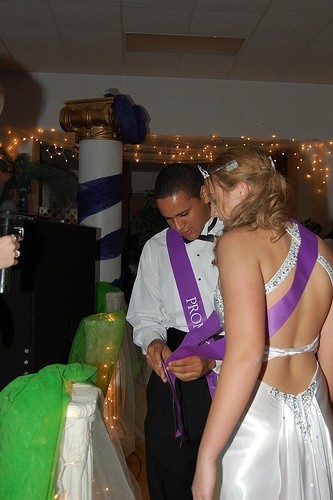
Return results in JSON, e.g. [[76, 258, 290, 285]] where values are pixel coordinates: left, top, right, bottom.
[[0, 210, 101, 390]]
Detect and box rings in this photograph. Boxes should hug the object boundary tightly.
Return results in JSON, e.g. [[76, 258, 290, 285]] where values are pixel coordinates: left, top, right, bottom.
[[12, 234, 19, 241], [15, 250, 21, 258]]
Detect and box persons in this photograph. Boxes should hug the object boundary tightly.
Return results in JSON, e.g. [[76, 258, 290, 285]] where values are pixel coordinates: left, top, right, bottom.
[[0, 233, 21, 269], [123, 159, 235, 500], [189, 146, 333, 500]]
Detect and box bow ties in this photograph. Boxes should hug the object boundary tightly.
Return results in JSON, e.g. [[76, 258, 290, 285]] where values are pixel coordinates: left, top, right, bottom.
[[183, 233, 213, 244]]
